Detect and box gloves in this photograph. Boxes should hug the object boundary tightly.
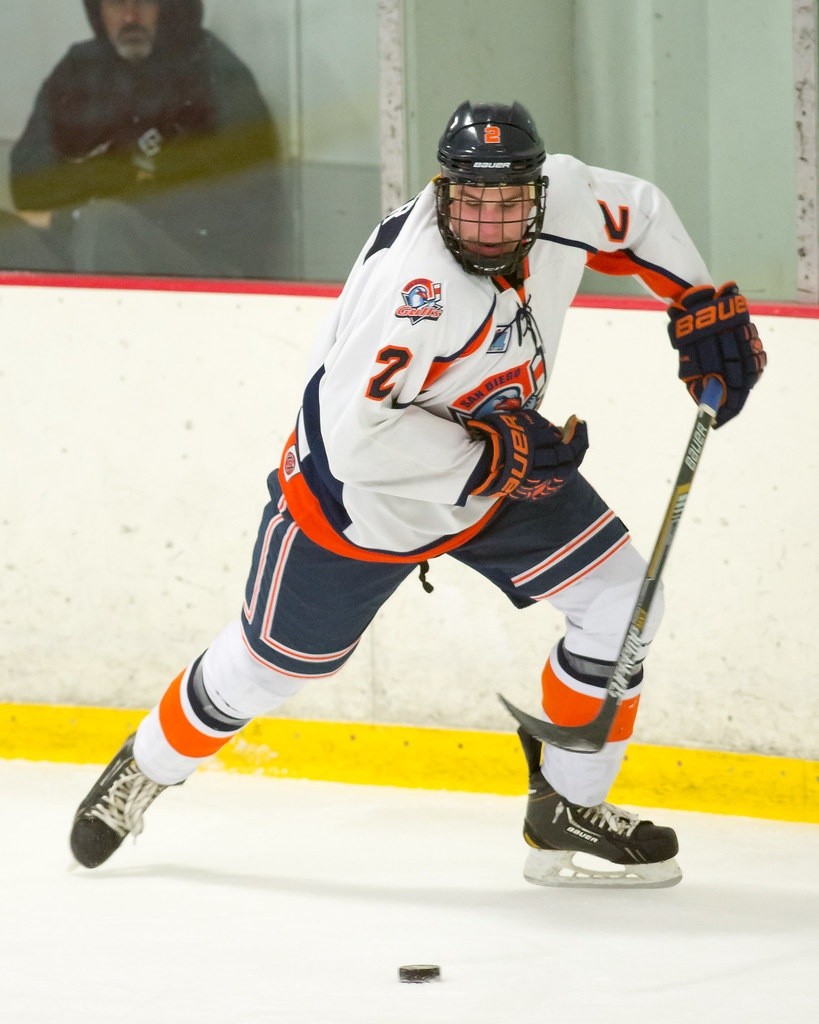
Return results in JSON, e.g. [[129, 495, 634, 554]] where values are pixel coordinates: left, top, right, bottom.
[[467, 409, 589, 503], [667, 280, 767, 431]]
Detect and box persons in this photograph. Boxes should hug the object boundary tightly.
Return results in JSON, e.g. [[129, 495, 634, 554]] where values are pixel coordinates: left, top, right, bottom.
[[70, 98, 769, 890], [1, 0, 293, 279]]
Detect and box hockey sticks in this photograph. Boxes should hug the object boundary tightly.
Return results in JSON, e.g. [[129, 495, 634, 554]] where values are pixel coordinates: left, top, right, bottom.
[[495, 368, 726, 758]]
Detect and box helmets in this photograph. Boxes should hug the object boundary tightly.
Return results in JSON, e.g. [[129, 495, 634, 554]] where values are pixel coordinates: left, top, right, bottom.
[[434, 100, 549, 276]]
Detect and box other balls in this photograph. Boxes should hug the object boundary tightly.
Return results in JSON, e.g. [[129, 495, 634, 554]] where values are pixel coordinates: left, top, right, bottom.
[[398, 964, 441, 985]]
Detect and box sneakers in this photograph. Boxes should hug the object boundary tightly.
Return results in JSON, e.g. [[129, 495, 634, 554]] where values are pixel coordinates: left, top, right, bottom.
[[70, 730, 187, 869], [517, 724, 683, 889]]
